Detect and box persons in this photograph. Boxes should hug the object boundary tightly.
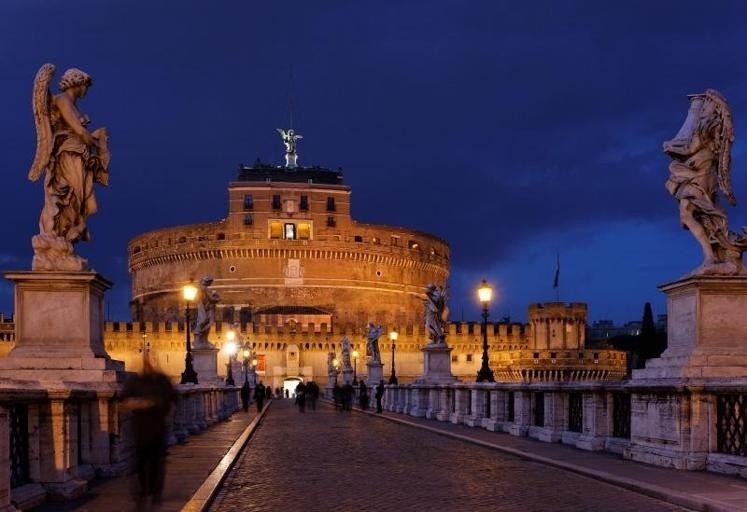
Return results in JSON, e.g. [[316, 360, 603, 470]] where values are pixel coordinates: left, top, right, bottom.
[[240, 381, 250, 411], [190, 275, 220, 348], [366, 321, 383, 363], [254, 380, 264, 413], [661, 89, 745, 274], [30, 67, 111, 270], [117, 350, 180, 508], [282, 129, 297, 153], [340, 335, 351, 368], [266, 379, 386, 414], [423, 283, 450, 344]]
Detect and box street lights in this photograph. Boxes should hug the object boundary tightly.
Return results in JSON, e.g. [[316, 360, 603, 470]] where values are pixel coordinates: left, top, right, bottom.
[[388, 327, 399, 385], [475, 279, 497, 383], [243, 345, 250, 388], [142, 330, 147, 357], [251, 357, 257, 372], [226, 327, 235, 386], [351, 350, 359, 385], [180, 276, 200, 385]]
